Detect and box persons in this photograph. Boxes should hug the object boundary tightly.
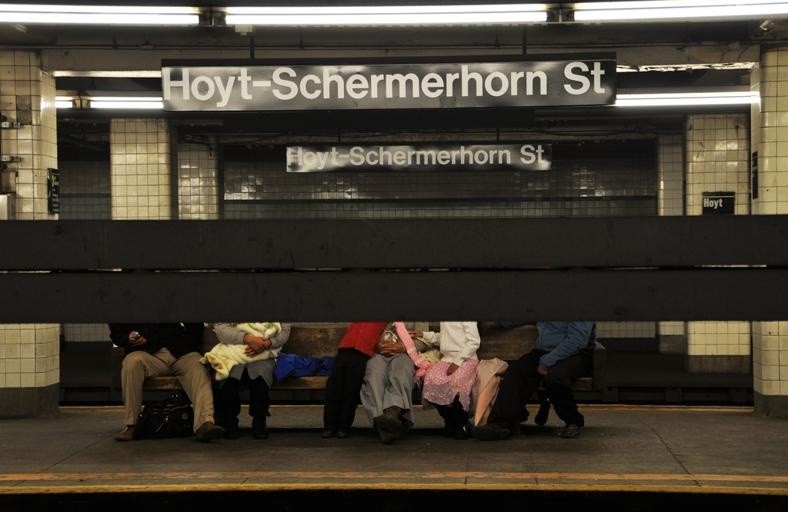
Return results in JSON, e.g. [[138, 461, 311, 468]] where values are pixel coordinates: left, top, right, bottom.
[[321, 322, 394, 440], [406, 321, 481, 441], [479, 320, 597, 440], [104, 320, 226, 442], [206, 321, 291, 440], [360, 322, 431, 445]]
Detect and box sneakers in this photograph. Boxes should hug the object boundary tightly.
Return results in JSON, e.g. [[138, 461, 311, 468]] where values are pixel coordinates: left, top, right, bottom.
[[114, 425, 136, 442], [533, 400, 551, 426], [252, 412, 270, 439], [223, 425, 240, 440], [555, 412, 585, 438], [196, 421, 225, 442], [445, 419, 502, 441], [321, 423, 353, 438], [372, 406, 407, 444]]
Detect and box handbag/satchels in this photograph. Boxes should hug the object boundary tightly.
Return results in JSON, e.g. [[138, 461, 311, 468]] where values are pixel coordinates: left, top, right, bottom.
[[374, 330, 407, 357], [133, 397, 197, 439]]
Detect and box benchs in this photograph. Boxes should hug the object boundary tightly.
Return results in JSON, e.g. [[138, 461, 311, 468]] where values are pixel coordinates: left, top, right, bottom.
[[112, 319, 593, 443]]
